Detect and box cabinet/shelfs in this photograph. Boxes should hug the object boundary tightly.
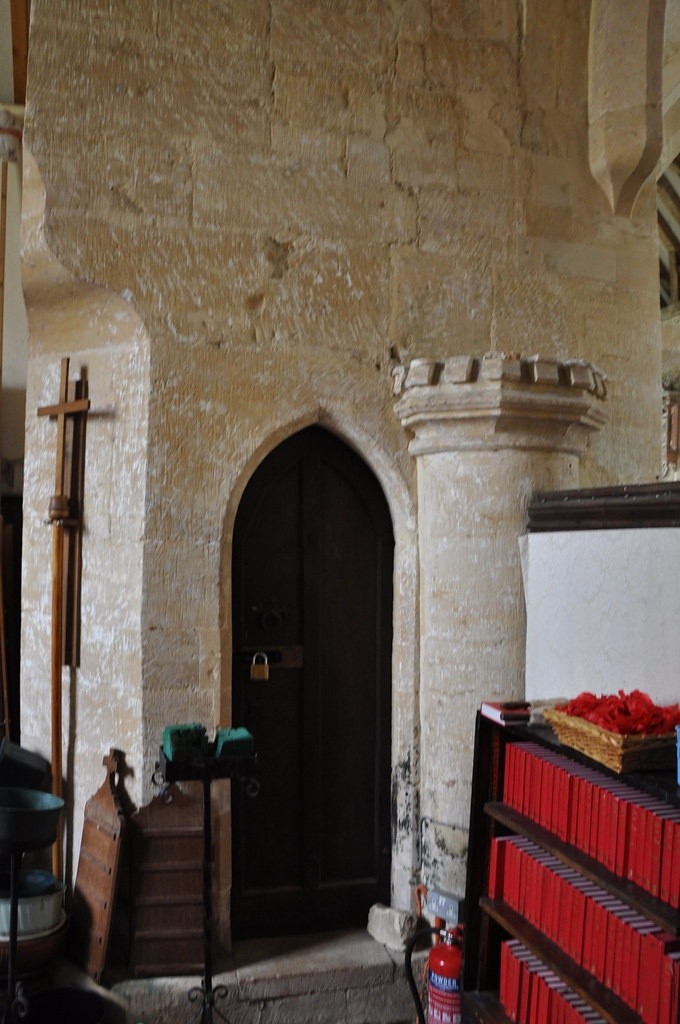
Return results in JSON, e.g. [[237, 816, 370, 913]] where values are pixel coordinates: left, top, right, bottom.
[[459, 710, 680, 1024]]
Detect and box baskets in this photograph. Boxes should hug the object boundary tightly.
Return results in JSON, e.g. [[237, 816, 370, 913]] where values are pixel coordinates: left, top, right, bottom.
[[543, 705, 676, 774]]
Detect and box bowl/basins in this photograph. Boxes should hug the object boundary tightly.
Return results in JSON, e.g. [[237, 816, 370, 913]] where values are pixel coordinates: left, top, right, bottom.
[[0, 786, 64, 850], [0, 868, 65, 941]]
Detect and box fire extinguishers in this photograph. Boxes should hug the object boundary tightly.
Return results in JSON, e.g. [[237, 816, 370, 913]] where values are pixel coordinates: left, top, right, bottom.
[[404, 925, 468, 1024]]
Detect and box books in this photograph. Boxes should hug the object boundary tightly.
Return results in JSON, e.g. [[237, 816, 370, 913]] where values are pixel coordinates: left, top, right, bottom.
[[479, 701, 531, 726], [490, 743, 680, 907], [488, 834, 680, 1024]]
